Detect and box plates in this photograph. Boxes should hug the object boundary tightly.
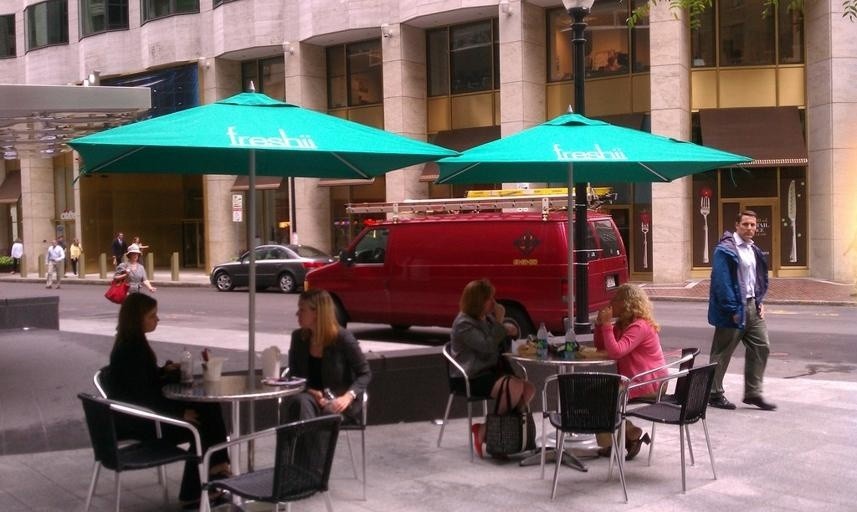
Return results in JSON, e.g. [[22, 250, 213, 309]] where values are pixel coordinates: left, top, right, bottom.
[[260, 376, 307, 386]]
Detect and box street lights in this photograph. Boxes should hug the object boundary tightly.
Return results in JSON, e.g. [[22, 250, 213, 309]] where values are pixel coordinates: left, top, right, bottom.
[[556, 0, 601, 339]]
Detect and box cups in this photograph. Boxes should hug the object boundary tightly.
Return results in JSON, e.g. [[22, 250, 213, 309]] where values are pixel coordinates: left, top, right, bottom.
[[200, 356, 225, 380]]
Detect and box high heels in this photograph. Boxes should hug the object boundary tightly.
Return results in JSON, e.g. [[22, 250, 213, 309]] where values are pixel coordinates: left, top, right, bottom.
[[598, 431, 651, 460]]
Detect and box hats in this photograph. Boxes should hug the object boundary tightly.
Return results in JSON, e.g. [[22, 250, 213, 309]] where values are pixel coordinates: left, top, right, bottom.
[[126, 244, 142, 257]]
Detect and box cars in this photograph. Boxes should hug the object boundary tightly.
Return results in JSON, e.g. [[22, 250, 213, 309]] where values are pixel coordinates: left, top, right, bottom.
[[209, 241, 338, 295]]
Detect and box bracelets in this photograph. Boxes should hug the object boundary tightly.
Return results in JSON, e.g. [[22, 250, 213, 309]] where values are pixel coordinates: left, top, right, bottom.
[[349, 389, 356, 400]]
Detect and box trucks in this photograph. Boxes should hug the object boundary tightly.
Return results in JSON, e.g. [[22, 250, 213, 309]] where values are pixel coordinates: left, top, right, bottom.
[[302, 190, 639, 346]]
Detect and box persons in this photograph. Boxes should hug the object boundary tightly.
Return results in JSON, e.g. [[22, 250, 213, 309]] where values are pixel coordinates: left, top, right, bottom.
[[106, 291, 236, 511], [593, 282, 671, 462], [285, 286, 372, 425], [10, 231, 158, 303], [447, 278, 537, 461], [705, 210, 778, 411]]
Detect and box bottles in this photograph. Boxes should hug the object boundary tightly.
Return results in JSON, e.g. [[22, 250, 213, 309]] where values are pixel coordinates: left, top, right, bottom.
[[179, 347, 194, 385], [258, 344, 280, 382], [564, 327, 577, 359], [537, 320, 548, 358], [324, 388, 346, 423]]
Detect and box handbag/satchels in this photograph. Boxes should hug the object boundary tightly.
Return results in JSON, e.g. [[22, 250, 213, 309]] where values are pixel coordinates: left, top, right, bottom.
[[105, 270, 131, 304], [487, 412, 537, 454]]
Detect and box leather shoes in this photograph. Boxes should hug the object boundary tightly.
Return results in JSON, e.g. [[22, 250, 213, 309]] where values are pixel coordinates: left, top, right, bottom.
[[743, 396, 776, 410], [710, 397, 736, 409], [472, 424, 483, 459]]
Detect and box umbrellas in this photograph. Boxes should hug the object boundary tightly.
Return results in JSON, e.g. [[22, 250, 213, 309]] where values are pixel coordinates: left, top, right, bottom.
[[430, 100, 754, 356], [61, 77, 463, 371]]
